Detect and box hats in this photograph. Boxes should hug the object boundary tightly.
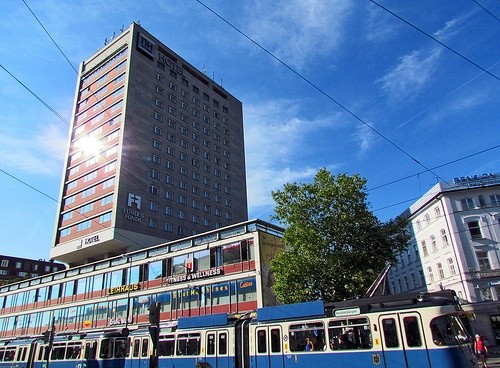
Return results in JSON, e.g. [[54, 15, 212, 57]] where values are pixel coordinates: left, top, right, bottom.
[[474, 333, 481, 338]]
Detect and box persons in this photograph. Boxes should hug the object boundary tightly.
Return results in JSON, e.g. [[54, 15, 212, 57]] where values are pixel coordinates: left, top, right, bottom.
[[474, 333, 489, 368], [290, 329, 372, 350], [0, 338, 216, 359]]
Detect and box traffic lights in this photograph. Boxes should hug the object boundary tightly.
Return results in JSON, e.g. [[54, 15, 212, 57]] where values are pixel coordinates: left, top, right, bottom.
[[42, 329, 51, 345], [148, 302, 161, 349]]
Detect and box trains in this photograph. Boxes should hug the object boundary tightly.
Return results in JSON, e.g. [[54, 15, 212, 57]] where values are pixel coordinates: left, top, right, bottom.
[[0, 284, 479, 368]]
